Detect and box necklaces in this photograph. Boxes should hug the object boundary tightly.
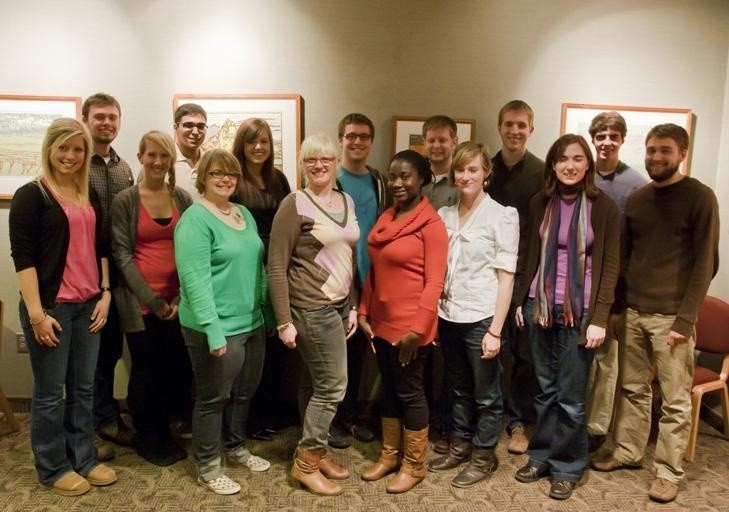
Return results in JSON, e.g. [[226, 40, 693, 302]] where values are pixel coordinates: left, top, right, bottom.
[[308, 188, 335, 207], [204, 194, 233, 218]]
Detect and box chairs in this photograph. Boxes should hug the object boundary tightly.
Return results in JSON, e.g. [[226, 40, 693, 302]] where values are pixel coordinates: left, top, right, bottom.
[[686, 295, 729, 462]]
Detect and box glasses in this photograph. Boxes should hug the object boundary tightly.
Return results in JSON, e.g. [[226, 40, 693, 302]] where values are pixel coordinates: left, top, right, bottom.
[[342, 131, 371, 139], [208, 170, 238, 180], [176, 121, 209, 130]]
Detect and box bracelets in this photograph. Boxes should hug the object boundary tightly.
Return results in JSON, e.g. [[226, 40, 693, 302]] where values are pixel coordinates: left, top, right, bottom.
[[488, 329, 503, 342], [28, 309, 48, 327], [275, 320, 293, 330], [101, 285, 111, 293], [349, 305, 359, 313]]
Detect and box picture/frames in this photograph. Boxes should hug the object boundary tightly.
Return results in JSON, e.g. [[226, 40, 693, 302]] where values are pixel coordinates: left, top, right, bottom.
[[391, 115, 476, 165], [560, 103, 693, 177], [170, 93, 303, 207], [0, 93, 82, 203]]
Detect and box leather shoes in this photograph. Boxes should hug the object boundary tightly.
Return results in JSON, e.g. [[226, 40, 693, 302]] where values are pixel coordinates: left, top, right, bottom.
[[515, 463, 541, 483], [250, 430, 274, 442], [547, 480, 576, 500], [263, 427, 281, 435], [645, 475, 681, 503], [589, 450, 644, 474]]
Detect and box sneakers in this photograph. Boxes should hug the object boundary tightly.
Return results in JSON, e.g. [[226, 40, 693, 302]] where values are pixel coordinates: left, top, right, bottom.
[[197, 471, 242, 495], [221, 446, 271, 472], [341, 419, 375, 443], [38, 469, 90, 497], [328, 423, 351, 449], [92, 441, 117, 462], [430, 430, 451, 454], [157, 419, 198, 466], [85, 463, 118, 488], [507, 427, 529, 455], [94, 415, 138, 447], [588, 434, 607, 451], [134, 438, 176, 467]]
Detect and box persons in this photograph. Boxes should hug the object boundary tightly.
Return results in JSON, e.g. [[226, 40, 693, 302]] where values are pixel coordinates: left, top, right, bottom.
[[107, 128, 195, 468], [8, 116, 117, 497], [589, 119, 721, 505], [509, 133, 622, 502], [224, 117, 293, 444], [580, 109, 651, 455], [169, 150, 271, 498], [424, 142, 524, 489], [133, 102, 210, 442], [476, 98, 552, 456], [263, 131, 364, 496], [410, 113, 460, 455], [324, 113, 396, 450], [78, 92, 140, 465], [355, 147, 450, 499]]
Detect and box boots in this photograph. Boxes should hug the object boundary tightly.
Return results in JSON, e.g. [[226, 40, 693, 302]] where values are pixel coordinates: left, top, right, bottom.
[[290, 444, 344, 496], [427, 439, 473, 472], [360, 415, 405, 482], [385, 423, 431, 494], [450, 442, 500, 489], [312, 447, 351, 481]]
